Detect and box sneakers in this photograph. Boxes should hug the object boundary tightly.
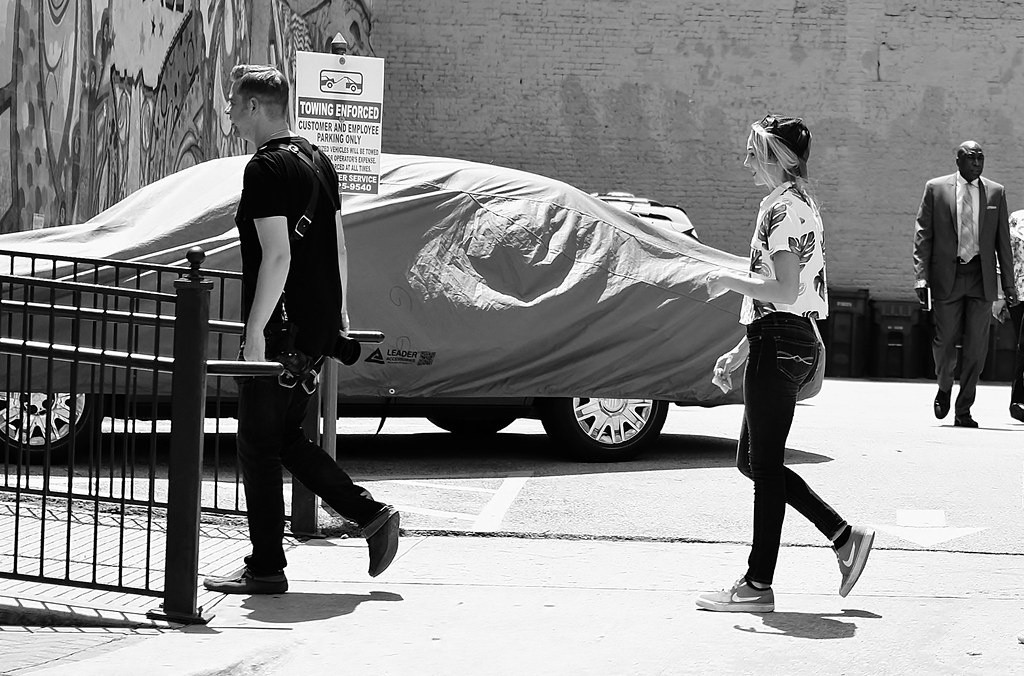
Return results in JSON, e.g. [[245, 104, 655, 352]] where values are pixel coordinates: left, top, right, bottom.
[[831, 526, 875, 598], [695, 577, 775, 612]]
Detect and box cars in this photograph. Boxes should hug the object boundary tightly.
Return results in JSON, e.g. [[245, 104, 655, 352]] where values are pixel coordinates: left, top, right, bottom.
[[0, 153, 827, 462]]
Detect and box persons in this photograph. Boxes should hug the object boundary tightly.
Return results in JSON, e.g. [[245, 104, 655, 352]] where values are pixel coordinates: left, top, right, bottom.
[[912, 138, 1019, 428], [694, 112, 877, 615], [202, 64, 401, 596], [994, 208, 1024, 423]]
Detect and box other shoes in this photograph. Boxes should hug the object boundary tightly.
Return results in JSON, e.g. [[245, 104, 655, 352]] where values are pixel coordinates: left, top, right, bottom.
[[361, 506, 400, 577], [934, 386, 952, 419], [1009, 402, 1024, 422], [203, 568, 288, 594], [954, 416, 978, 428]]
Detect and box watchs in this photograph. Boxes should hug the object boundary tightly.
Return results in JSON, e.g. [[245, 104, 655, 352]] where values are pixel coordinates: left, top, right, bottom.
[[997, 295, 1006, 300]]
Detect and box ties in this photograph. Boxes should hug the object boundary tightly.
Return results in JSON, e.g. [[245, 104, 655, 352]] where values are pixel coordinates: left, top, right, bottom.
[[960, 185, 974, 263]]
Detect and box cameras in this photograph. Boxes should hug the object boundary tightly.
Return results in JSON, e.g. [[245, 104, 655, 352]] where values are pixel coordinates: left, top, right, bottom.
[[294, 304, 362, 365]]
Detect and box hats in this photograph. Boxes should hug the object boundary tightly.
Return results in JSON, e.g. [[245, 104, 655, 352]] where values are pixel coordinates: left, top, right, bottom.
[[761, 115, 812, 181]]
